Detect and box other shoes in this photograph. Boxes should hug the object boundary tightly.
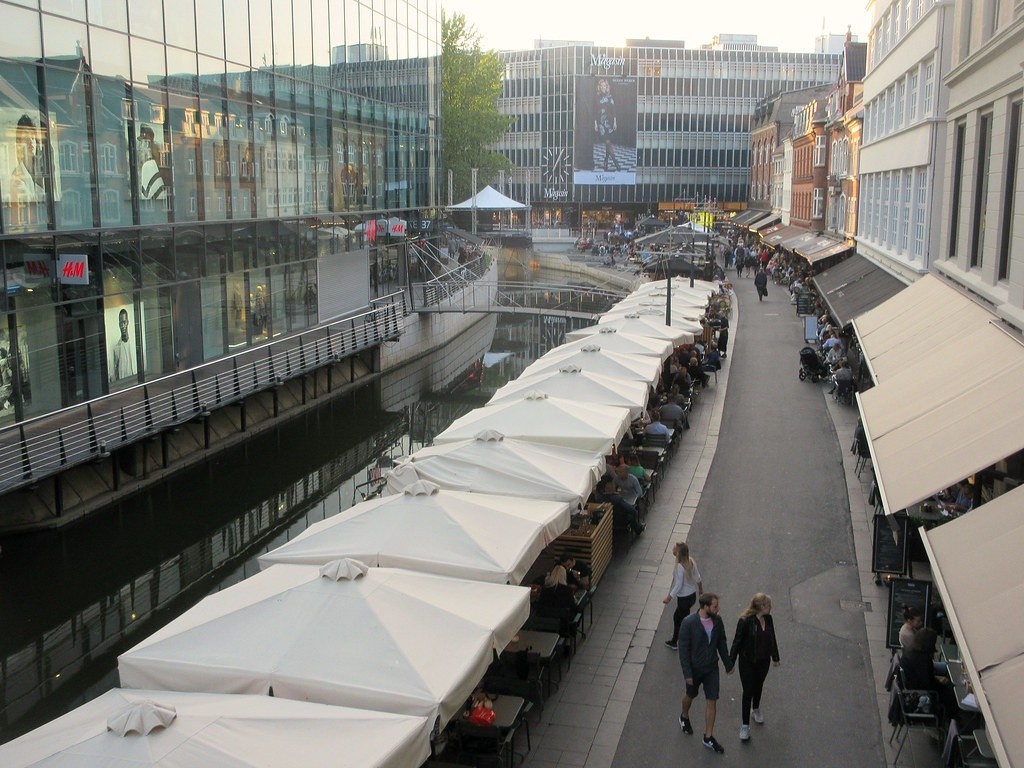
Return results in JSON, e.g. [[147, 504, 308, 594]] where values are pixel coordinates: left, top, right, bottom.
[[702, 376, 709, 386], [636, 522, 647, 536], [664, 640, 678, 650], [739, 725, 751, 739], [751, 708, 764, 723]]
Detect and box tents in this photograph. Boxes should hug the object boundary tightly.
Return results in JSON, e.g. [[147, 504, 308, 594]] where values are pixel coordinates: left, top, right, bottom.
[[446, 185, 528, 236], [540, 326, 673, 368], [256, 479, 571, 587], [486, 363, 649, 421], [565, 313, 694, 349], [432, 389, 632, 457], [608, 299, 710, 317], [600, 308, 704, 337], [519, 343, 662, 393], [625, 275, 718, 300], [385, 427, 606, 515], [0, 564, 531, 768]]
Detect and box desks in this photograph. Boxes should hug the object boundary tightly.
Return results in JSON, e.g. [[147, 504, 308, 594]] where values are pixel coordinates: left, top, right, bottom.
[[618, 492, 640, 541], [458, 693, 524, 768], [643, 446, 665, 479], [946, 664, 966, 685], [574, 589, 593, 632], [906, 500, 941, 520], [645, 468, 655, 503], [954, 686, 980, 733], [960, 729, 994, 758], [458, 364, 994, 768], [668, 429, 675, 457], [504, 630, 561, 696], [939, 643, 960, 662]]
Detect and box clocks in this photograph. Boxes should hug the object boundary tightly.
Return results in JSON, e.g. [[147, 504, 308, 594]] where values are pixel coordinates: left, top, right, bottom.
[[541, 146, 572, 185]]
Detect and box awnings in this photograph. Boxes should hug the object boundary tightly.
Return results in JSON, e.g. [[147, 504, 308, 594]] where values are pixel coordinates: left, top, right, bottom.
[[917, 484, 1024, 768], [781, 231, 817, 252], [849, 271, 999, 386], [734, 206, 785, 237], [794, 235, 850, 265], [854, 319, 1024, 516], [811, 253, 909, 331], [760, 226, 808, 249]]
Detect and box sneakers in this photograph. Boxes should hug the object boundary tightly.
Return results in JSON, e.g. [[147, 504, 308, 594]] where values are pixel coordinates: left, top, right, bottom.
[[702, 734, 724, 754], [678, 713, 693, 735]]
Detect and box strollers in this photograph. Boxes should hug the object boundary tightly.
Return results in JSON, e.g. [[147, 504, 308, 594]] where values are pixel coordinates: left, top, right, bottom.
[[799, 346, 828, 383]]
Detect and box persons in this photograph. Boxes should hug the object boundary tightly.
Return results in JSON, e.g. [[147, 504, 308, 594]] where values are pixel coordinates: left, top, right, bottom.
[[10, 116, 43, 229], [446, 238, 478, 271], [594, 79, 621, 172], [535, 308, 723, 645], [137, 123, 170, 200], [678, 593, 729, 755], [730, 593, 780, 740], [724, 237, 993, 748], [663, 543, 704, 650], [109, 309, 138, 381]]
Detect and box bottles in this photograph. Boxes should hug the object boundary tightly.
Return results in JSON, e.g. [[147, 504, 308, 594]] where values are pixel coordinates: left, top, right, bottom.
[[544, 572, 551, 585]]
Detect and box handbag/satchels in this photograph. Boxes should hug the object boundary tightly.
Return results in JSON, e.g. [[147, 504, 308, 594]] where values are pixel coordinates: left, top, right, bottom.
[[763, 289, 768, 296]]
